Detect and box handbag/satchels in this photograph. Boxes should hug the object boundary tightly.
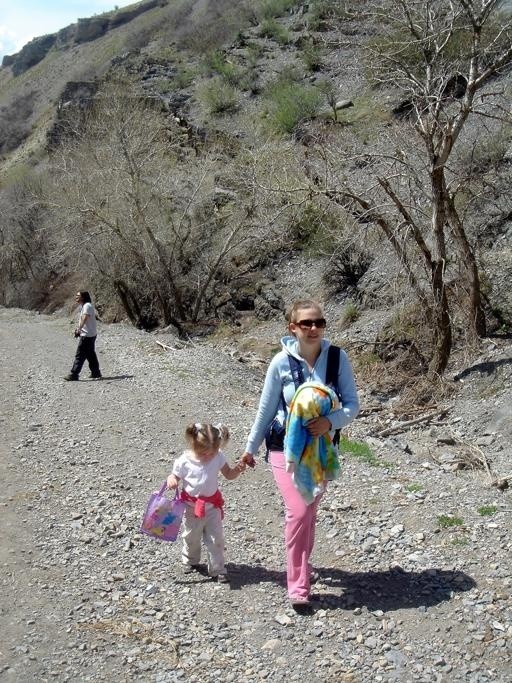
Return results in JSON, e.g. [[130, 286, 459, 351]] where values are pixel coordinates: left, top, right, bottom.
[[141, 480, 187, 542]]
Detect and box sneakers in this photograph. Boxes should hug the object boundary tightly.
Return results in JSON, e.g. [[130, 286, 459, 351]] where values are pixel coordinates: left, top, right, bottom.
[[212, 573, 230, 582], [291, 598, 309, 605], [183, 564, 194, 573], [63, 373, 79, 381], [88, 373, 101, 378]]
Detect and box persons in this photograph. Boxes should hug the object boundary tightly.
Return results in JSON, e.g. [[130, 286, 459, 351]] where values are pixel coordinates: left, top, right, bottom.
[[62, 290, 102, 381], [166, 422, 246, 583], [239, 299, 361, 613]]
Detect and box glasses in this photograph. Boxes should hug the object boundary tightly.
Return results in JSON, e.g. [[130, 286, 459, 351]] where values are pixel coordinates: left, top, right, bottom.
[[294, 319, 326, 328]]
